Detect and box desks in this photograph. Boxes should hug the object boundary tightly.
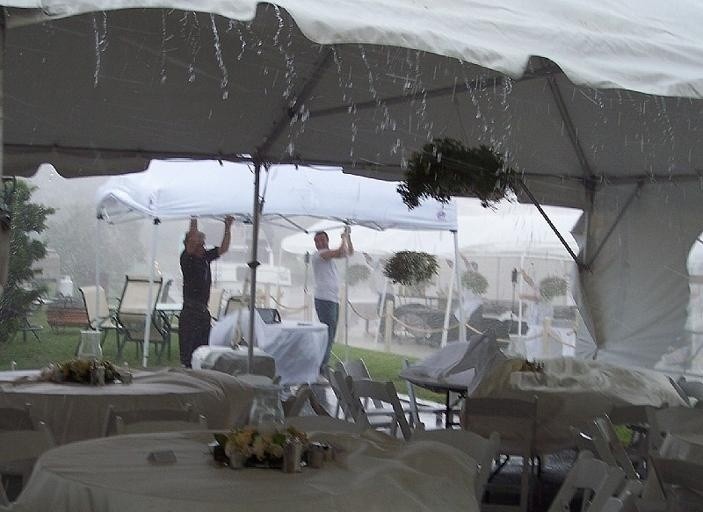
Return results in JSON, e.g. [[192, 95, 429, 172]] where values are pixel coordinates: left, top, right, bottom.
[[188, 312, 329, 388]]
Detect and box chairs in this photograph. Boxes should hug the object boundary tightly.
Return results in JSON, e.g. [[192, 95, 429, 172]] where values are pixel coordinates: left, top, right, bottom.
[[1, 353, 703, 512], [72, 272, 253, 358]]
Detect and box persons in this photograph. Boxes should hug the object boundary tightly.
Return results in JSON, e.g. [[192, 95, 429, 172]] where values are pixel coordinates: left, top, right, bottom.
[[313, 230, 354, 375], [179, 214, 236, 369]]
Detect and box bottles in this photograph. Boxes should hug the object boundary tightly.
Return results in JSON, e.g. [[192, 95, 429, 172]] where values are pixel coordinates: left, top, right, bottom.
[[78, 330, 103, 363], [249, 384, 285, 438]]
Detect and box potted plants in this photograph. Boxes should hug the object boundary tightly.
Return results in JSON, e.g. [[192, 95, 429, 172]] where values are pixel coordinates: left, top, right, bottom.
[[395, 135, 521, 216]]
